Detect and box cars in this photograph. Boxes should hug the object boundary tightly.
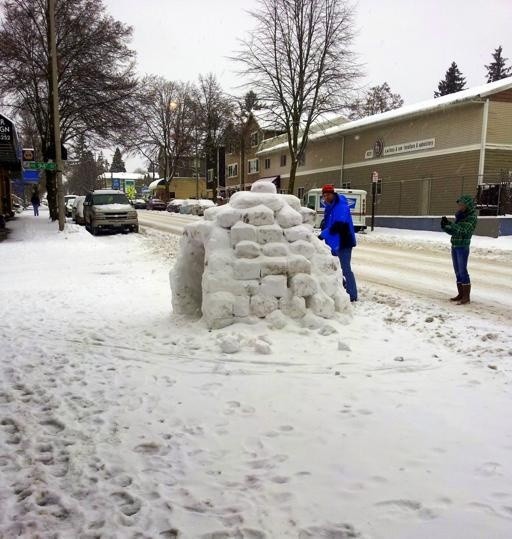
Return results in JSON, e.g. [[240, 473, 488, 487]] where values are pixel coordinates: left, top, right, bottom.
[[63, 188, 214, 238]]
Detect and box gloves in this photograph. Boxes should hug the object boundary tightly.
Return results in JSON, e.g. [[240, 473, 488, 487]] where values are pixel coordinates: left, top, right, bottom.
[[441, 217, 452, 228]]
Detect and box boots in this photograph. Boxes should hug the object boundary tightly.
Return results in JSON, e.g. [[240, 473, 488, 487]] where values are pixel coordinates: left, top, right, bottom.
[[457, 283, 471, 305], [450, 282, 462, 302]]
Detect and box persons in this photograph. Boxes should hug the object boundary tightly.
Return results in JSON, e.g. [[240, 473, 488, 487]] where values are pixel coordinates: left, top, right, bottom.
[[31, 192, 41, 217], [439, 194, 479, 305], [317, 184, 357, 302]]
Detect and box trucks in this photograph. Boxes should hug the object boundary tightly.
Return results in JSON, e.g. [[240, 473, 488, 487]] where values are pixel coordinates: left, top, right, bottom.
[[303, 188, 367, 236]]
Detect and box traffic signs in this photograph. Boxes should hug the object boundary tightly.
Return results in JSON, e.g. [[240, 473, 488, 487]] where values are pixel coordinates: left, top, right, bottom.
[[30, 162, 56, 170]]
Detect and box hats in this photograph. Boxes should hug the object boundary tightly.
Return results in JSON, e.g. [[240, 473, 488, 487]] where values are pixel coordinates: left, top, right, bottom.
[[321, 184, 335, 193]]
